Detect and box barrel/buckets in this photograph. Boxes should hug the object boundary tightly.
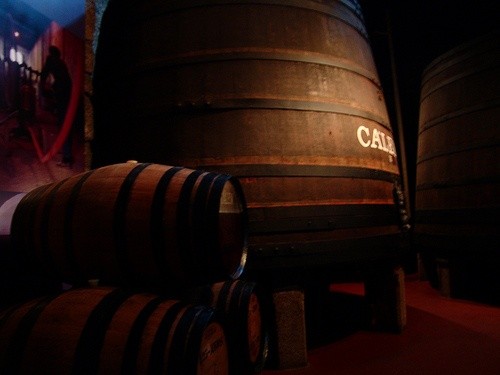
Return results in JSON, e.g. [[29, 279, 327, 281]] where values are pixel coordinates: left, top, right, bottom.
[[9, 162, 248, 292], [93, 0, 414, 336], [181, 279, 277, 375], [411, 34, 500, 298], [0, 287, 239, 375]]
